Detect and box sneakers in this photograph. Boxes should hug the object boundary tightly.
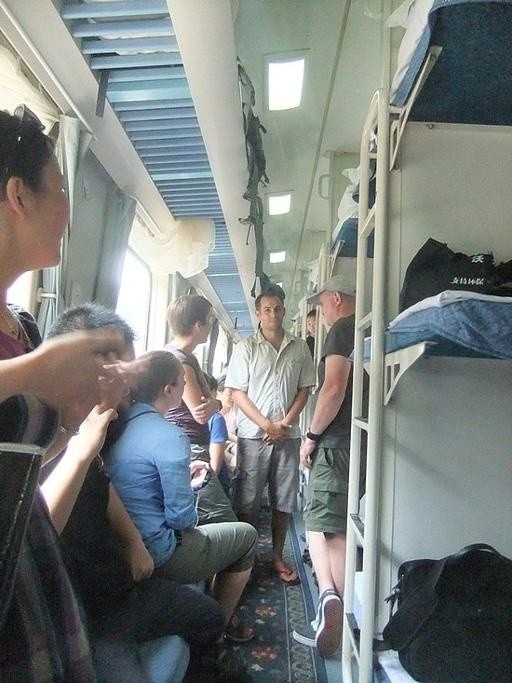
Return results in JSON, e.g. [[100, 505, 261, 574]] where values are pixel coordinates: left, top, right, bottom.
[[200, 650, 248, 677], [293, 620, 316, 648], [315, 590, 342, 657]]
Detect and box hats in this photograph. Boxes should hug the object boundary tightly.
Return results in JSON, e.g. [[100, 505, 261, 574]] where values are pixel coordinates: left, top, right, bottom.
[[305, 274, 356, 305]]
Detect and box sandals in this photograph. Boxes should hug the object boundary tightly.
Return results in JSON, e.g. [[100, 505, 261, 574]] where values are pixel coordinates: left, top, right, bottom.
[[225, 615, 255, 642]]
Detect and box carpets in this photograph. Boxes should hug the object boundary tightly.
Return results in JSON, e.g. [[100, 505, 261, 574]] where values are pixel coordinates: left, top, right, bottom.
[[182, 506, 391, 683]]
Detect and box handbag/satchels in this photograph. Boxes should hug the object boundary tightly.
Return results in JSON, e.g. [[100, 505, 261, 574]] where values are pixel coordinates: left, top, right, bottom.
[[383, 543, 510, 683], [400, 237, 512, 314]]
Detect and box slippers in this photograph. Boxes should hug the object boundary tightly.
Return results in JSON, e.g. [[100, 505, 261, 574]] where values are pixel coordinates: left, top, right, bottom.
[[276, 569, 300, 585]]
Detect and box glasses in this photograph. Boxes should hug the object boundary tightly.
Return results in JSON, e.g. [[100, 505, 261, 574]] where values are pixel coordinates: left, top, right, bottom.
[[3, 104, 45, 185]]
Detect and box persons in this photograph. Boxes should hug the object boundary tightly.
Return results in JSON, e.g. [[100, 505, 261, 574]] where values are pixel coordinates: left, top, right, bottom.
[[0, 107, 126, 683], [38, 273, 368, 683]]
